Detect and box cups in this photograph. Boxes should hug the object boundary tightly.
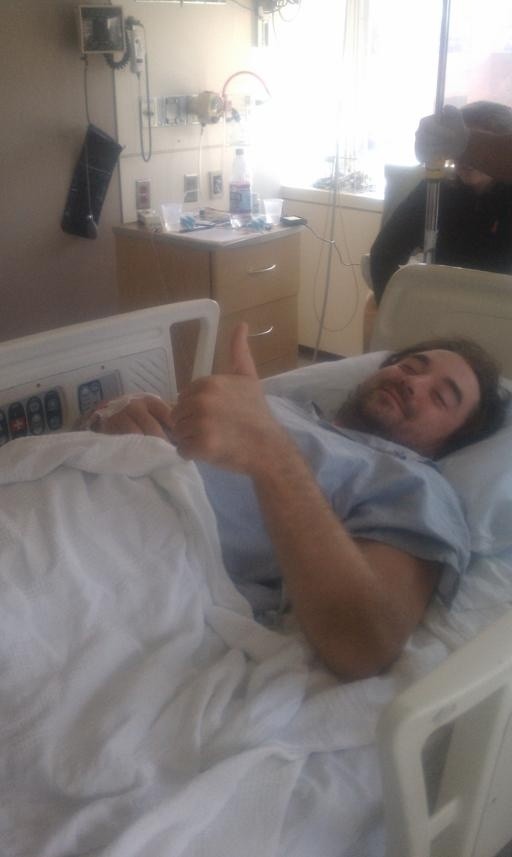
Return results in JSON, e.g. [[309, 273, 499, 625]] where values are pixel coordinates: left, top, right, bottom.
[[264, 199, 284, 226], [161, 203, 181, 232]]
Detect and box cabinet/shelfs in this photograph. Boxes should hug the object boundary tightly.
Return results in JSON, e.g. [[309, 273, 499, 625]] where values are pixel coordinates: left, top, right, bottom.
[[114, 232, 300, 385]]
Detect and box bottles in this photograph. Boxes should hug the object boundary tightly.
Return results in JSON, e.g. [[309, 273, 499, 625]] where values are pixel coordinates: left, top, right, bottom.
[[228, 148, 252, 228]]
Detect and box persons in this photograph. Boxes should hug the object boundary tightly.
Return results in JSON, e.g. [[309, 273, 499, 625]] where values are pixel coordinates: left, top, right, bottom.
[[369, 101, 510, 306], [415, 104, 510, 179], [73, 320, 508, 683]]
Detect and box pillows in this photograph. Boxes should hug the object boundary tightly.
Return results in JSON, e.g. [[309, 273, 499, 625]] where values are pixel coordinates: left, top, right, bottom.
[[261, 342, 512, 558]]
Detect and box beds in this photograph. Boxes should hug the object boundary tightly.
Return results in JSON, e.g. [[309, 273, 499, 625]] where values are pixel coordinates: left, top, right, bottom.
[[0, 261, 512, 857]]
